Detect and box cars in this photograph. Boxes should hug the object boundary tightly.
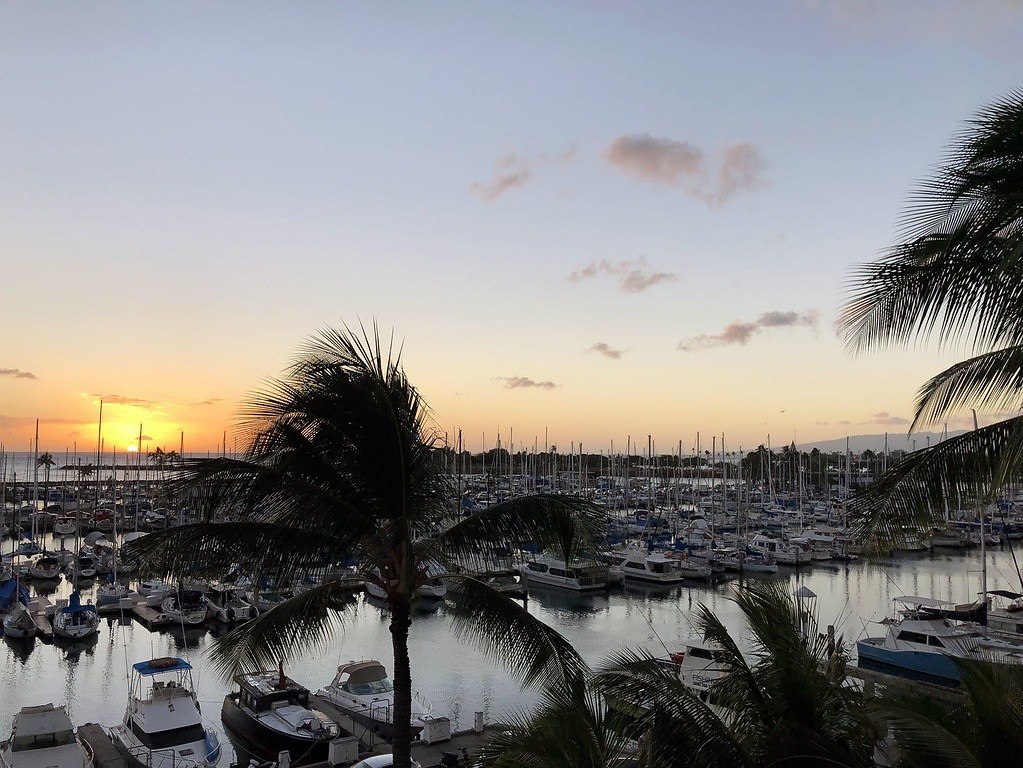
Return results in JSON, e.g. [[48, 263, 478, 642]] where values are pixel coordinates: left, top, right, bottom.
[[349, 754, 422, 768]]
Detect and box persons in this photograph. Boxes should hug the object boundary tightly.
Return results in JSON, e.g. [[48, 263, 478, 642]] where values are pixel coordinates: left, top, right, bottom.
[[278, 743, 291, 768]]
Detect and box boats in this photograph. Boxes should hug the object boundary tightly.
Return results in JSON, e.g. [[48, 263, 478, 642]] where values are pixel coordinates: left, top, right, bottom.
[[222, 661, 341, 764], [310, 656, 432, 743], [599, 627, 736, 731], [857, 565, 1023, 682], [0, 703, 94, 768], [108, 594, 221, 768]]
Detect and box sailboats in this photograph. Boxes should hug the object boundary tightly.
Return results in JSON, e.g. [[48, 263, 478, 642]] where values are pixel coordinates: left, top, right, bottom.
[[955, 409, 1023, 632], [0, 400, 1023, 640]]
